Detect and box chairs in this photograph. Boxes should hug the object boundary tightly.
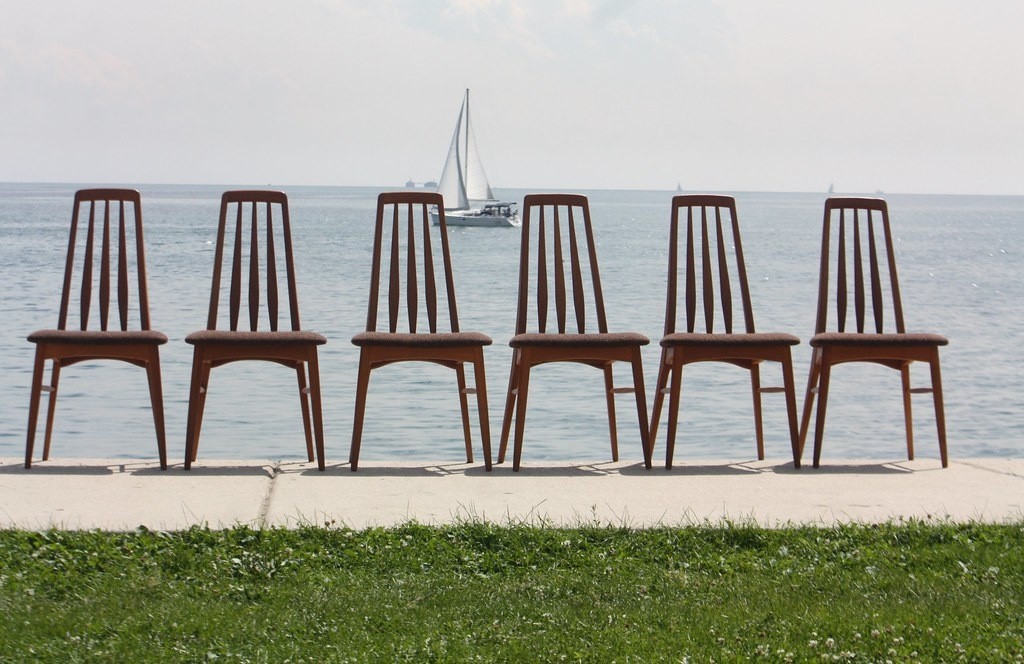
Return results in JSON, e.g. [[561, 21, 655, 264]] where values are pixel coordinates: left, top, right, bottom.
[[498, 193, 650, 470], [20, 189, 168, 470], [349, 192, 493, 472], [649, 196, 801, 468], [801, 198, 949, 468], [185, 190, 327, 471]]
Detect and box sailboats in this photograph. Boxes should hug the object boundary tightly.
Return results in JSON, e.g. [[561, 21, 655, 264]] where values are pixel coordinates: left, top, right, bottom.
[[428, 88, 522, 227]]
